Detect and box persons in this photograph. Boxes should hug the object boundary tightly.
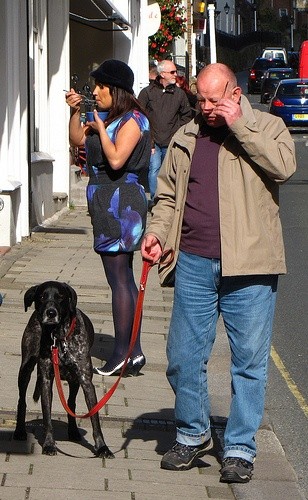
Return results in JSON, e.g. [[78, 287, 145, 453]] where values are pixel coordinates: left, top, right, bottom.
[[141, 64, 297, 483], [64, 59, 150, 377], [137, 59, 196, 200]]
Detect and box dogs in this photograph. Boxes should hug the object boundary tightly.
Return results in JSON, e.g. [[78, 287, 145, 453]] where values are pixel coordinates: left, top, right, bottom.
[[16, 280, 116, 459]]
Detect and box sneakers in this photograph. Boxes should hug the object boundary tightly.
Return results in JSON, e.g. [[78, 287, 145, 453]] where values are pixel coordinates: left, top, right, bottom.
[[160, 436, 214, 471], [219, 457, 255, 483]]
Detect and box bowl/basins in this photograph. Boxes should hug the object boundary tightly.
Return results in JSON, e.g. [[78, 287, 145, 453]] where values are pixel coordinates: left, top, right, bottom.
[[85, 112, 109, 121]]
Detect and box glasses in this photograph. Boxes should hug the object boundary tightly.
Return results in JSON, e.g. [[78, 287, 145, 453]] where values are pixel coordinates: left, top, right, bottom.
[[164, 69, 178, 75], [185, 81, 229, 115]]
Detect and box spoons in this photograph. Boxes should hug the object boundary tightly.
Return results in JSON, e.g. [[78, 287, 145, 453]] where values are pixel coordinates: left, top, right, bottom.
[[62, 90, 96, 104]]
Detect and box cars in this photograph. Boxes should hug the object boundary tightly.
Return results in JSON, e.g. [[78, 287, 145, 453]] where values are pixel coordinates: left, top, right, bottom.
[[246, 47, 299, 93], [257, 67, 297, 103], [263, 79, 308, 132]]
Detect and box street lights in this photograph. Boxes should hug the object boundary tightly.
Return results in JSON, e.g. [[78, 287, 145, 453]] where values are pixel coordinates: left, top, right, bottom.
[[288, 17, 294, 52], [224, 2, 231, 34], [252, 3, 258, 33]]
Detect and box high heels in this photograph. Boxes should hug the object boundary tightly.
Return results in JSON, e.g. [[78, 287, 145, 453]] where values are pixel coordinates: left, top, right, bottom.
[[92, 353, 146, 378]]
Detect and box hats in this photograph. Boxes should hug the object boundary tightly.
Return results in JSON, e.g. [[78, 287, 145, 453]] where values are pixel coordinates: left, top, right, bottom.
[[90, 60, 135, 95]]
